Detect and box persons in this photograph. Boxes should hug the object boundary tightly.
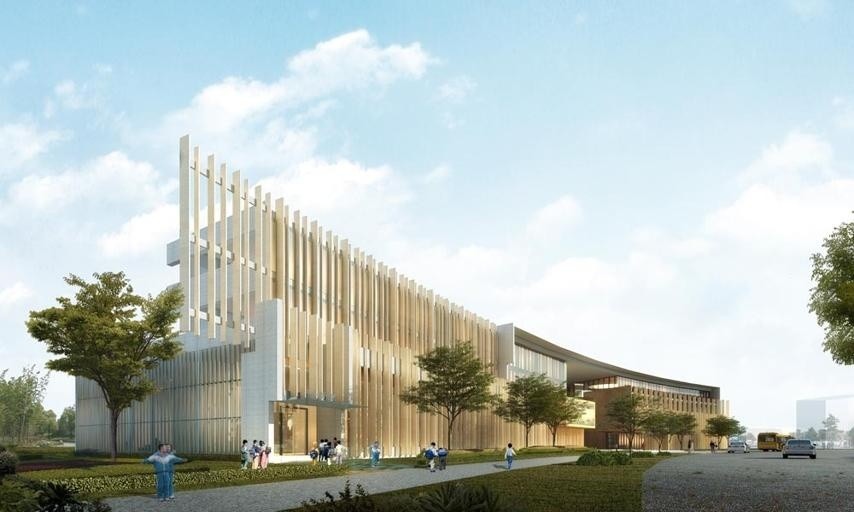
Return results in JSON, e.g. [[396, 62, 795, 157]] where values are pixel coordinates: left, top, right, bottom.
[[505, 442, 517, 469], [367, 441, 381, 468], [240, 436, 348, 472], [144, 442, 191, 501], [424, 442, 438, 472], [709, 439, 716, 455], [436, 447, 449, 471], [143, 444, 185, 502]]
